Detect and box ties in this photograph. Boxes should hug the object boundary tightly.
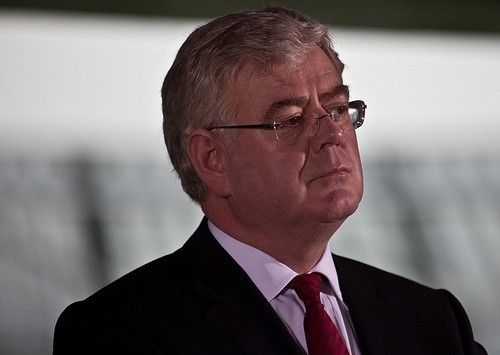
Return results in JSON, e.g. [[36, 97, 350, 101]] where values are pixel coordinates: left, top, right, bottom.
[[287, 272, 350, 354]]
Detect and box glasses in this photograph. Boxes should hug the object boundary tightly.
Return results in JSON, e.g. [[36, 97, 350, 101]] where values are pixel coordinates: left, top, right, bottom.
[[206, 99, 366, 145]]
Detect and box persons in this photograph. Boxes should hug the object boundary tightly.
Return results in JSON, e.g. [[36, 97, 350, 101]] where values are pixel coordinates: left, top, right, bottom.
[[53, 6, 490, 355]]
